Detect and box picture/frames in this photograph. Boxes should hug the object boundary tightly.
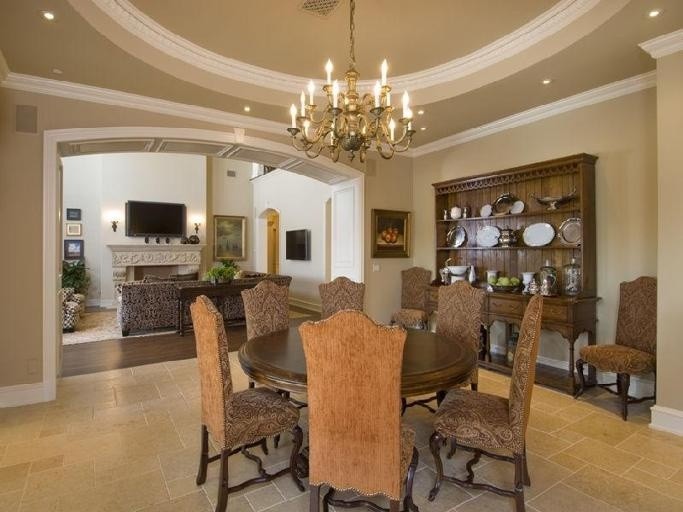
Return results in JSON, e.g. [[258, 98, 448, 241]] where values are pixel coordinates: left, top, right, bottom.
[[371, 209, 412, 258], [66, 223, 81, 235], [67, 208, 81, 220], [64, 240, 84, 260], [213, 215, 247, 261]]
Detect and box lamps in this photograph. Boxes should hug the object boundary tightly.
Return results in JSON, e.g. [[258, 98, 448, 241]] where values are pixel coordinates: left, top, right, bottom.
[[111, 215, 119, 232], [287, 0, 416, 163], [193, 218, 202, 235]]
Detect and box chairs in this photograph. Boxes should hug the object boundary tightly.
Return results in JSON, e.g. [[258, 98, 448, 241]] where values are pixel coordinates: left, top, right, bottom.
[[575, 276, 657, 421], [63, 293, 80, 333], [318, 276, 365, 316], [428, 294, 543, 512], [62, 288, 85, 318], [390, 266, 434, 331], [241, 280, 308, 447], [435, 280, 486, 391], [298, 310, 418, 512], [189, 295, 306, 512]]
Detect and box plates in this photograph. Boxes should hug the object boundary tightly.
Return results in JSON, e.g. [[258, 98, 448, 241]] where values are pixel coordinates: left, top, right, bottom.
[[521, 222, 555, 247], [510, 201, 524, 213], [446, 226, 465, 248], [476, 226, 500, 246], [558, 218, 581, 247], [491, 192, 513, 217], [479, 203, 491, 217]]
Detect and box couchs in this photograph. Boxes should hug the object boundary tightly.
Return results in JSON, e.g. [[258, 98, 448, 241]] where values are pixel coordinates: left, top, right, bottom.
[[117, 269, 292, 336]]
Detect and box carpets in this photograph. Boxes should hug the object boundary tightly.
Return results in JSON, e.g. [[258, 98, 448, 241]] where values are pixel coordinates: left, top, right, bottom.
[[62, 306, 178, 346]]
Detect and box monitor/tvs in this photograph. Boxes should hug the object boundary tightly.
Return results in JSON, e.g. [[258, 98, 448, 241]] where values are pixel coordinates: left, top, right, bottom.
[[286, 229, 308, 260], [127, 201, 185, 237]]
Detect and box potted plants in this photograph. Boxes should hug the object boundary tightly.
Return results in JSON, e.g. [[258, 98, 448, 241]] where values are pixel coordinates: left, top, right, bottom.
[[208, 267, 234, 284]]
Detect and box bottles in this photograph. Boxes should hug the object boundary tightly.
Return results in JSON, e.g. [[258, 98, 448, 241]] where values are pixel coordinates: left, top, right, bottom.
[[504, 332, 519, 367], [441, 204, 469, 220], [496, 225, 516, 247], [381, 226, 400, 243], [478, 324, 485, 355], [448, 260, 580, 297]]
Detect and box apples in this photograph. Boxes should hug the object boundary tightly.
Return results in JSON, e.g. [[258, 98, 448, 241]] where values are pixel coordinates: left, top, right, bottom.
[[381, 227, 399, 242]]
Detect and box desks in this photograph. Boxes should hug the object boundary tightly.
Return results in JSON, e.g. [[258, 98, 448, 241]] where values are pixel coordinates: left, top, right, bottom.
[[176, 282, 258, 336], [238, 326, 479, 478]]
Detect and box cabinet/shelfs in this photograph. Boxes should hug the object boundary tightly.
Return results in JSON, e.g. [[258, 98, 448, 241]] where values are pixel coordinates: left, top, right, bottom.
[[430, 154, 602, 398]]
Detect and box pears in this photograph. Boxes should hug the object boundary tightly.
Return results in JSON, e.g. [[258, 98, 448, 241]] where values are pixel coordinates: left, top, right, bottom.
[[488, 275, 520, 287]]
